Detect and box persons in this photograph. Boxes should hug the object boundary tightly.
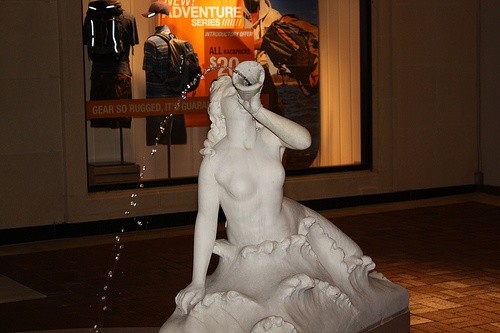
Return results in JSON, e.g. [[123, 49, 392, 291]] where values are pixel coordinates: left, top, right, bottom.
[[141, 1, 188, 146], [241, 0, 284, 76], [175, 61, 364, 316], [83, 0, 140, 128]]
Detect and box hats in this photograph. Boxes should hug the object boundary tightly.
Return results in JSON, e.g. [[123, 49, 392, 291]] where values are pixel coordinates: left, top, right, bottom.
[[142, 2, 169, 17]]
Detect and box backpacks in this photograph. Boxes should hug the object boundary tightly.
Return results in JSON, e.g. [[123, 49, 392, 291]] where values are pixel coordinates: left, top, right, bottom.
[[261, 15, 319, 94], [142, 35, 203, 93]]
